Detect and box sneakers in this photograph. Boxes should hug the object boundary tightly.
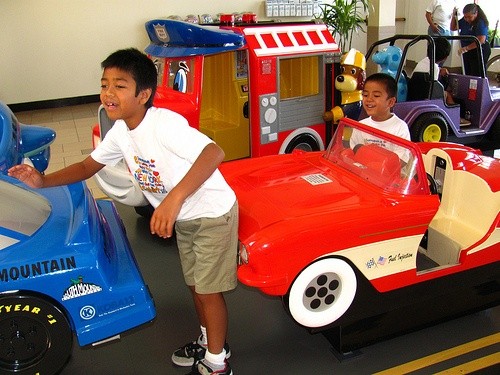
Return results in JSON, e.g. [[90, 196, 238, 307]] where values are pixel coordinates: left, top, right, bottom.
[[187, 359, 233, 375], [171, 333, 232, 366]]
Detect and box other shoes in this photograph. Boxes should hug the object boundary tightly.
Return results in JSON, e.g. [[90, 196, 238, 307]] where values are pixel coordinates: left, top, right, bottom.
[[460, 119, 471, 127]]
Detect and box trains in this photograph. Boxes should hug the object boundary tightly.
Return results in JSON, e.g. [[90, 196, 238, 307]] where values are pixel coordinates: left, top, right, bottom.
[[93, 20, 500, 146]]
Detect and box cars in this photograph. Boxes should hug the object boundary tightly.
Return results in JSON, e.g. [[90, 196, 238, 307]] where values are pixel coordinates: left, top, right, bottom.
[[1, 104, 156, 373]]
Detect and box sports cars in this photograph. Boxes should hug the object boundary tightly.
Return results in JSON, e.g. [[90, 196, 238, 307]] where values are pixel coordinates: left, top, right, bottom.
[[216, 120, 499, 348]]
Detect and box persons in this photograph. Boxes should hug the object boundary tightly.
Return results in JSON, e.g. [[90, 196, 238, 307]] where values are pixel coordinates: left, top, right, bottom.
[[450, 4, 491, 75], [426, 0, 456, 67], [349, 73, 411, 169], [7, 47, 240, 375], [411, 39, 472, 126]]
